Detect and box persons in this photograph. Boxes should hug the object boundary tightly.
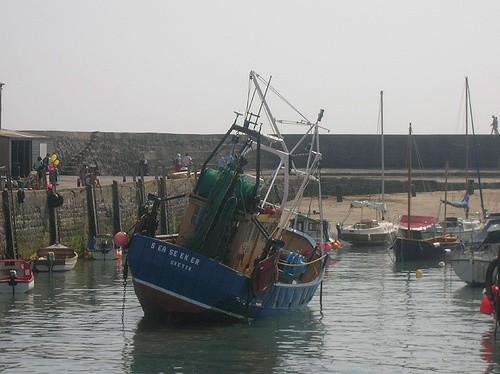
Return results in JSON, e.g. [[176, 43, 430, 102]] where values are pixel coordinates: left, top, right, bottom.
[[173, 153, 182, 173], [183, 153, 193, 174], [35, 154, 59, 185], [490, 116, 499, 135]]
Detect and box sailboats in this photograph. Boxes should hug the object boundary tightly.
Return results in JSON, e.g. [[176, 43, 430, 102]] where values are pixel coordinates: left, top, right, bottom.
[[336, 90, 398, 248], [391, 77, 500, 286]]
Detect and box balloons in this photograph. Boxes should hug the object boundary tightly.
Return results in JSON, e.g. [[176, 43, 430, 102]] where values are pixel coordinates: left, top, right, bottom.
[[52, 154, 59, 165]]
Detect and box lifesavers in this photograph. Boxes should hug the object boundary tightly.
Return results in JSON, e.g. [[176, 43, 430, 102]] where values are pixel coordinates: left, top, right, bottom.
[[18, 188, 26, 203]]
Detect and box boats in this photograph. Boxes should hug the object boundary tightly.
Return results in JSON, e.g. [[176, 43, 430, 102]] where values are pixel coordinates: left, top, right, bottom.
[[28, 242, 79, 272], [289, 212, 331, 251], [126, 70, 340, 324], [91, 234, 123, 260], [0, 259, 35, 295]]
